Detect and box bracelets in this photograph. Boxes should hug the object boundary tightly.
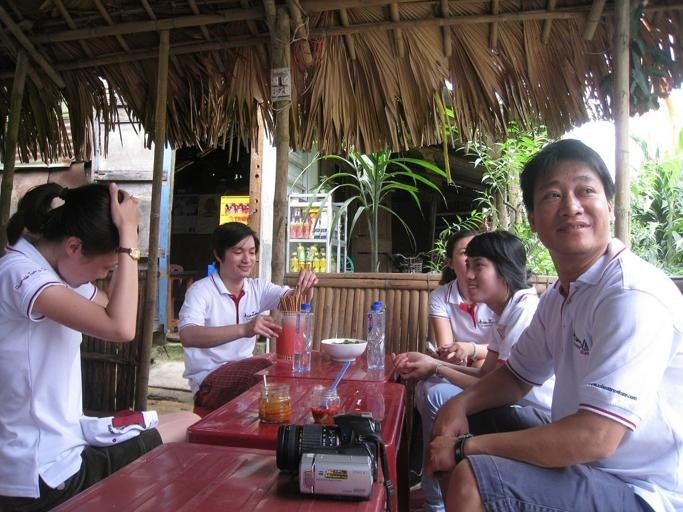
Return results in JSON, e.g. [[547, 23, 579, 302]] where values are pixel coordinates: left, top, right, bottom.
[[469, 341, 478, 360]]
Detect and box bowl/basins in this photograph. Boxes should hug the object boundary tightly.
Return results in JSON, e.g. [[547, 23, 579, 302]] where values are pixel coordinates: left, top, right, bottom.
[[319, 337, 368, 362]]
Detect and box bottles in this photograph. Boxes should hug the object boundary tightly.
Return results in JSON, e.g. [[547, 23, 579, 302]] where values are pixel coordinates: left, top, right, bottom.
[[290, 208, 326, 240], [258, 384, 291, 423], [368, 305, 384, 369], [311, 387, 342, 423], [293, 304, 314, 372], [291, 242, 325, 272]]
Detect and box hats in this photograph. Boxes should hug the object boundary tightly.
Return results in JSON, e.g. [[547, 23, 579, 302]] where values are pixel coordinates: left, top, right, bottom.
[[76, 407, 160, 448]]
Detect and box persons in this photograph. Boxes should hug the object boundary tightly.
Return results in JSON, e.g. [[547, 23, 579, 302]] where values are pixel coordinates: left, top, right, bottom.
[[0, 182, 202, 512], [427, 228, 499, 366], [178, 222, 320, 410], [424, 139, 683, 512], [390, 231, 554, 512]]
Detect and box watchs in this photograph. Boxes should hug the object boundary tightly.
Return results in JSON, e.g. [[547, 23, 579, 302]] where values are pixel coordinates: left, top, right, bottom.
[[454, 431, 473, 464], [435, 364, 445, 377], [115, 246, 141, 260]]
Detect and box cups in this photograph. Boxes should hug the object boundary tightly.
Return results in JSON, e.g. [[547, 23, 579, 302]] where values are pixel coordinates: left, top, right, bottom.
[[266, 311, 294, 361]]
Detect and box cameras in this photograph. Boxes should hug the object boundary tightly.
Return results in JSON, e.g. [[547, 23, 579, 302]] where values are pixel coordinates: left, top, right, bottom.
[[276, 411, 381, 502]]
